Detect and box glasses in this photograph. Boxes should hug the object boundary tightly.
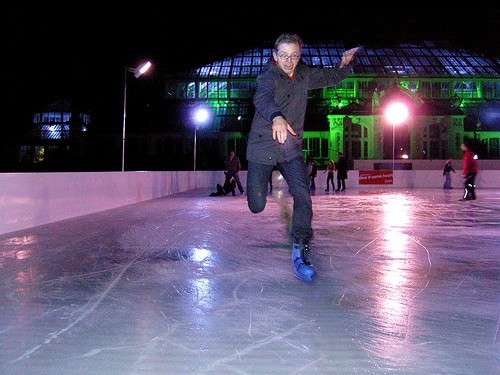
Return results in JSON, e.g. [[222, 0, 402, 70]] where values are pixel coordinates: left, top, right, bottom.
[[276, 53, 300, 60]]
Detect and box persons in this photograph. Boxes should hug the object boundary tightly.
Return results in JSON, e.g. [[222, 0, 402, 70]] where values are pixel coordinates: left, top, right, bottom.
[[209, 169, 237, 197], [458, 143, 480, 201], [442, 161, 456, 189], [321, 160, 336, 192], [225, 149, 244, 197], [306, 161, 317, 190], [268, 171, 273, 192], [334, 152, 349, 193], [372, 87, 380, 106], [246, 31, 358, 282]]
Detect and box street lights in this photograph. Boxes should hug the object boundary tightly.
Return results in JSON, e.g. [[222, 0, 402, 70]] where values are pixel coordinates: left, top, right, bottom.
[[121, 60, 153, 171], [388, 102, 402, 170], [192, 109, 209, 172]]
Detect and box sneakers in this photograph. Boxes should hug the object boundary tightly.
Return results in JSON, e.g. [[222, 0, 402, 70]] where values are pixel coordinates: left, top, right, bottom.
[[335, 189, 340, 192], [341, 190, 346, 192], [292, 242, 316, 282], [325, 189, 329, 193]]
